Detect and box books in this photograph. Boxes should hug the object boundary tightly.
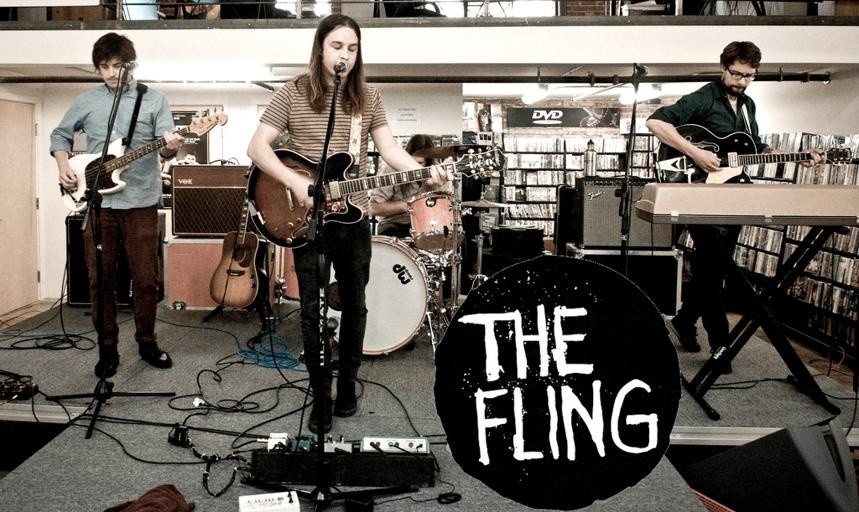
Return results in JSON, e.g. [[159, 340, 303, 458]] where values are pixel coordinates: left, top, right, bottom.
[[732, 132, 859, 345], [503, 136, 658, 236]]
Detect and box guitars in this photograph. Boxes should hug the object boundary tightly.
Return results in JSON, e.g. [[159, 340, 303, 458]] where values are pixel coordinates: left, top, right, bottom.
[[657, 124, 852, 183], [58, 108, 227, 212], [210, 163, 264, 308], [249, 149, 506, 248]]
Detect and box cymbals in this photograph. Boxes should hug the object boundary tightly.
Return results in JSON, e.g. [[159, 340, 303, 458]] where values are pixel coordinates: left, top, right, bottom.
[[459, 199, 510, 208]]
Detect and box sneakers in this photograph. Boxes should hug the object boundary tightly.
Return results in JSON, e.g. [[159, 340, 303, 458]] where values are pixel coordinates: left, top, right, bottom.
[[669, 317, 701, 352], [334, 379, 357, 417], [138, 342, 172, 369], [309, 395, 332, 433], [95, 352, 119, 378]]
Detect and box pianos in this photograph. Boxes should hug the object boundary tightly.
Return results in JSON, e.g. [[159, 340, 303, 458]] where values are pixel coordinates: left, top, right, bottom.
[[637, 184, 858, 226]]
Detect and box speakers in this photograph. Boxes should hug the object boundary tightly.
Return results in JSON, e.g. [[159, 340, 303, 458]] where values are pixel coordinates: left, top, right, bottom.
[[557, 176, 674, 249], [461, 228, 543, 296], [171, 163, 251, 238], [63, 210, 165, 309]]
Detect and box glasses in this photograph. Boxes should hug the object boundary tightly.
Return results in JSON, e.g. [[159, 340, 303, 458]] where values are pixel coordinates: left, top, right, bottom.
[[726, 67, 759, 81]]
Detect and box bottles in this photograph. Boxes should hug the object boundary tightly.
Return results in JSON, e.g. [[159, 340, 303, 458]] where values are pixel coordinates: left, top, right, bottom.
[[583, 139, 597, 177]]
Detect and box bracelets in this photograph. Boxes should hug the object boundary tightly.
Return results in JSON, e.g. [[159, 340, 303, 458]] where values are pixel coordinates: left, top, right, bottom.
[[159, 149, 180, 160]]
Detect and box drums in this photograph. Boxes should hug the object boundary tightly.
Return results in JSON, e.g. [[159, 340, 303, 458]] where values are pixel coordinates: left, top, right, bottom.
[[318, 235, 434, 357], [407, 190, 464, 248]]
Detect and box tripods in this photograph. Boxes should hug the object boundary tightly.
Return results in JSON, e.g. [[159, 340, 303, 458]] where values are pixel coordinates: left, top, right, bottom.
[[43, 70, 175, 442], [238, 73, 421, 512]]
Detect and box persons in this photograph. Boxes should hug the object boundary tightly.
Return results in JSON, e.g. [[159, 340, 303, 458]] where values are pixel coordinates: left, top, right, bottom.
[[644, 38, 824, 376], [366, 133, 437, 237], [245, 13, 456, 438], [50, 30, 184, 374]]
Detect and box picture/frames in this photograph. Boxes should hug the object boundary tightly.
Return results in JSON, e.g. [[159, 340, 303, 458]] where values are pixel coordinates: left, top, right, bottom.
[[161, 107, 212, 210]]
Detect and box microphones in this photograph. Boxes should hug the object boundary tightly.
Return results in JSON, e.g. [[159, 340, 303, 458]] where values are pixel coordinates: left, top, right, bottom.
[[635, 64, 649, 76], [121, 60, 138, 69], [333, 60, 348, 72]]
